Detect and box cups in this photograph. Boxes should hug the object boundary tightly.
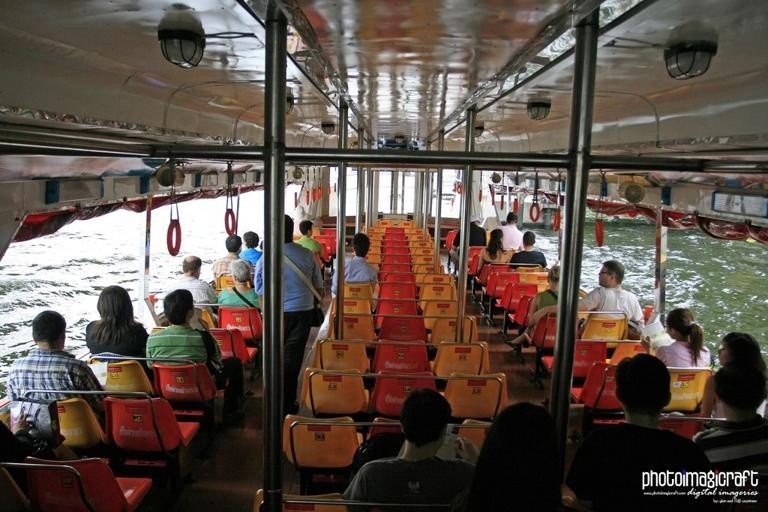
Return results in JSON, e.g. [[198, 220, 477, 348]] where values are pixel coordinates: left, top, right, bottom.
[[645, 306, 652, 325]]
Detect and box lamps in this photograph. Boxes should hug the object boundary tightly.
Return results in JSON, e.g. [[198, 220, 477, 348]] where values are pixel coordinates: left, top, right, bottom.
[[473, 127, 484, 137], [525, 102, 553, 121], [663, 40, 719, 82], [286, 96, 295, 113], [321, 124, 334, 135], [156, 24, 206, 71]]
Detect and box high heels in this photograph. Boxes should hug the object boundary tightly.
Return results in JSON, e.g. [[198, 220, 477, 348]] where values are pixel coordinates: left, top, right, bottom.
[[502, 339, 522, 352]]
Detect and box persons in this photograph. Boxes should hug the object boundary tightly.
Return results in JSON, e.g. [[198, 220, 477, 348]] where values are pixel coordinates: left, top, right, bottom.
[[640, 307, 712, 370], [452, 213, 548, 278], [566, 352, 716, 512], [0, 212, 327, 466], [699, 331, 767, 419], [532, 260, 643, 336], [341, 387, 476, 511], [502, 265, 560, 351], [331, 231, 379, 296], [691, 357, 768, 511], [450, 402, 583, 512]]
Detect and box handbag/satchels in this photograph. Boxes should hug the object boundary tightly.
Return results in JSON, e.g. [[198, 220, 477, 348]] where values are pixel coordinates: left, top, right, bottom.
[[10, 398, 66, 448], [310, 305, 324, 328]]
